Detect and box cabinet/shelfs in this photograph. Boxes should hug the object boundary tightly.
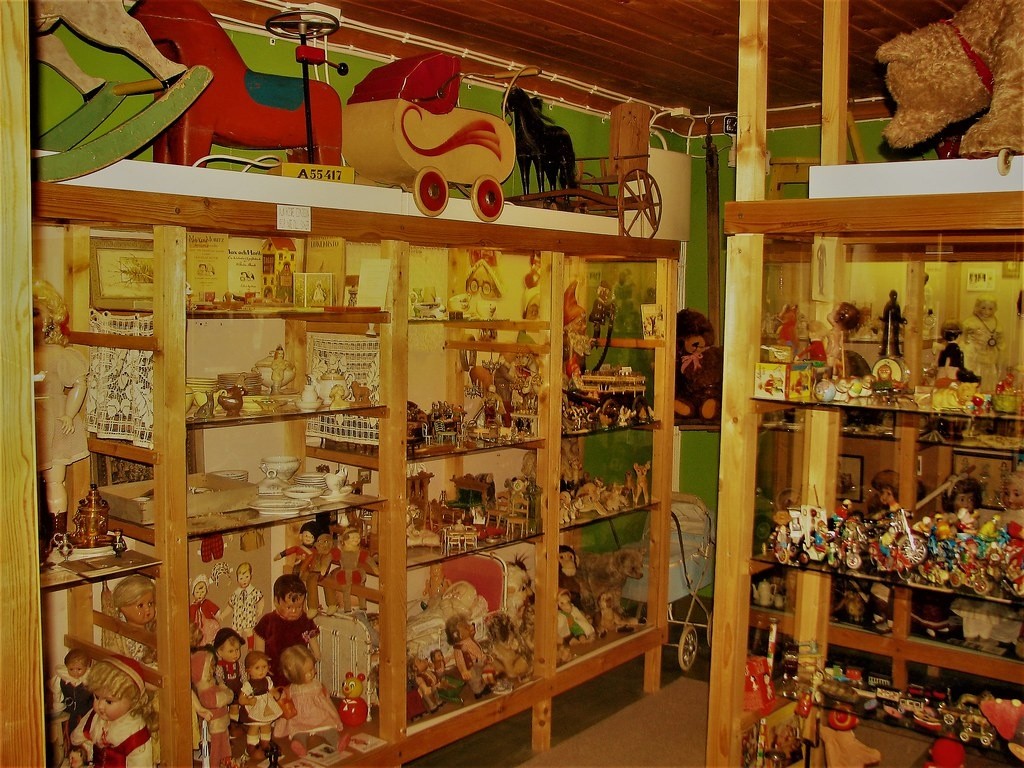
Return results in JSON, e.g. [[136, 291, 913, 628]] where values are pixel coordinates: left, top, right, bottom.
[[34, 180, 683, 768], [706, 0, 1024, 768], [429, 404, 466, 428], [509, 412, 538, 439]]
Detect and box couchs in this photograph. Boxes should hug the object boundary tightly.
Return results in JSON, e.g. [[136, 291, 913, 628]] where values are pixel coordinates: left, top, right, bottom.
[[407, 402, 428, 434]]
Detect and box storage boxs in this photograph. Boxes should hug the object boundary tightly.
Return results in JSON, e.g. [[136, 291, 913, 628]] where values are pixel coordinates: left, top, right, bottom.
[[754, 360, 814, 398], [293, 273, 333, 307], [804, 155, 1024, 200], [97, 469, 262, 527], [623, 148, 692, 242]]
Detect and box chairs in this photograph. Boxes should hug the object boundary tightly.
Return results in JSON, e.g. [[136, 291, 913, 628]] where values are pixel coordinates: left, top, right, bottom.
[[485, 491, 510, 529], [463, 534, 478, 552], [408, 430, 423, 458], [463, 526, 477, 547], [433, 419, 447, 443], [422, 423, 433, 448], [506, 496, 530, 538], [450, 525, 462, 547], [575, 101, 655, 240], [456, 423, 469, 446], [446, 534, 461, 556], [441, 529, 447, 555]]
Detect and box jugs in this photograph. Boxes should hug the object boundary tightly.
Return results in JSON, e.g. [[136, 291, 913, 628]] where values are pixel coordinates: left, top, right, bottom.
[[324, 465, 348, 496], [316, 368, 353, 406], [752, 579, 776, 607]]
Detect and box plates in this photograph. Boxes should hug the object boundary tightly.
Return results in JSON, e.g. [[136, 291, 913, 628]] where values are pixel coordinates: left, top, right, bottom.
[[211, 470, 249, 482], [247, 499, 310, 515], [186, 373, 264, 397], [282, 471, 327, 499], [320, 486, 352, 502]]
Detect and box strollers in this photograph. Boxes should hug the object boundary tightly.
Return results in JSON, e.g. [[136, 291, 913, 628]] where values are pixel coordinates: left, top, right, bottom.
[[621, 490, 715, 672]]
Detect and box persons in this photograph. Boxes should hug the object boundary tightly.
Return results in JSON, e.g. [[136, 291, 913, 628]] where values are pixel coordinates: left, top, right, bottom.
[[34, 258, 620, 767], [775, 293, 1024, 530]]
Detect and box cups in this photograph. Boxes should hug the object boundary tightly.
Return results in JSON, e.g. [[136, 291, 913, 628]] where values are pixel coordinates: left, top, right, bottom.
[[775, 594, 786, 608], [194, 390, 228, 408], [449, 293, 472, 313], [476, 302, 496, 318], [186, 393, 194, 415]]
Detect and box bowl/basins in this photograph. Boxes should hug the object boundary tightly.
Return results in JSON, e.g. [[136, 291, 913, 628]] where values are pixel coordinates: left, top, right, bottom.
[[260, 456, 301, 480], [49, 703, 67, 717], [294, 399, 324, 412]]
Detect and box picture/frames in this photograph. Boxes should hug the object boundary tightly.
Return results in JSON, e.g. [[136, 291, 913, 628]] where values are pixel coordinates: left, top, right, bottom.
[[89, 236, 153, 312], [967, 268, 996, 291], [832, 454, 864, 504], [357, 468, 372, 484], [1002, 261, 1019, 279], [947, 451, 1017, 513], [90, 429, 197, 485]]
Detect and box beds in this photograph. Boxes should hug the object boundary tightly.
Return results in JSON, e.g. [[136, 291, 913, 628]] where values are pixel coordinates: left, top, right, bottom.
[[466, 419, 499, 442], [313, 554, 508, 722]]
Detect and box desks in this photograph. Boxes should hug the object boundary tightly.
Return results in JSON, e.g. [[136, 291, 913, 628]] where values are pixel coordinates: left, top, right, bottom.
[[364, 520, 372, 544], [438, 431, 459, 445], [441, 507, 466, 522]]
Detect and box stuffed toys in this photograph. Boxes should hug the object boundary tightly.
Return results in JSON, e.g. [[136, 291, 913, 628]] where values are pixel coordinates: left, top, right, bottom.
[[672, 308, 724, 420], [876, 0, 1024, 175]]
